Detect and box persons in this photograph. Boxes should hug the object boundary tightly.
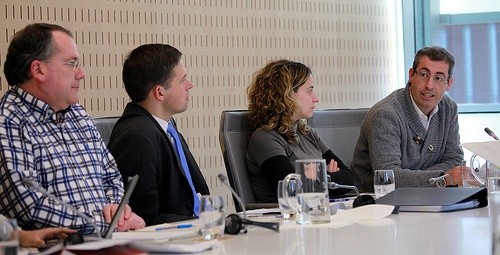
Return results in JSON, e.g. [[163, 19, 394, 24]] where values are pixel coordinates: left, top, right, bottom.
[[246, 60, 354, 203], [0, 23, 146, 242], [349, 46, 481, 187], [108, 44, 211, 227], [0, 213, 81, 246]]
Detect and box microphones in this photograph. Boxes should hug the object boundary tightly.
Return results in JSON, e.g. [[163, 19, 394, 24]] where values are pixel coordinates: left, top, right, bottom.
[[485, 127, 500, 140], [427, 173, 459, 188], [217, 173, 247, 220], [328, 182, 360, 196], [22, 177, 103, 243]]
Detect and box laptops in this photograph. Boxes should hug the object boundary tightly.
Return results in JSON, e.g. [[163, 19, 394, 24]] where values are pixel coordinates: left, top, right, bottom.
[[86, 175, 139, 240]]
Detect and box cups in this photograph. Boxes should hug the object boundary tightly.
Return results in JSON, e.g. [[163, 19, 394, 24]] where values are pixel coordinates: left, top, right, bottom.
[[282, 159, 331, 224], [278, 180, 297, 220], [199, 195, 226, 241], [470, 153, 500, 194], [374, 170, 395, 199], [462, 160, 480, 188]]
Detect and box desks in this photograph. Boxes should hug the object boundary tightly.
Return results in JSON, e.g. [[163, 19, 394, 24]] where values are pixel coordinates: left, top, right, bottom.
[[135, 193, 500, 255]]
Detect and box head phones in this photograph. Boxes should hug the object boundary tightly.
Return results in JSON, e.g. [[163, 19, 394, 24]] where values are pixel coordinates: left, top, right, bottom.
[[224, 214, 279, 236]]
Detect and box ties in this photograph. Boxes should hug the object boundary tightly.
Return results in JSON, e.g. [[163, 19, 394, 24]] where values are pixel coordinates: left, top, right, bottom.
[[166, 122, 201, 217]]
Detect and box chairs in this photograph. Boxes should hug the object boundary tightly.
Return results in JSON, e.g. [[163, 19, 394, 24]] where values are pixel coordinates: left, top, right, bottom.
[[219, 110, 254, 214], [305, 107, 372, 170]]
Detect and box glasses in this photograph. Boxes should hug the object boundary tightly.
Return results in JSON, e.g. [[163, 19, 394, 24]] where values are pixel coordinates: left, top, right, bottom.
[[414, 69, 450, 84], [43, 60, 82, 72]]
